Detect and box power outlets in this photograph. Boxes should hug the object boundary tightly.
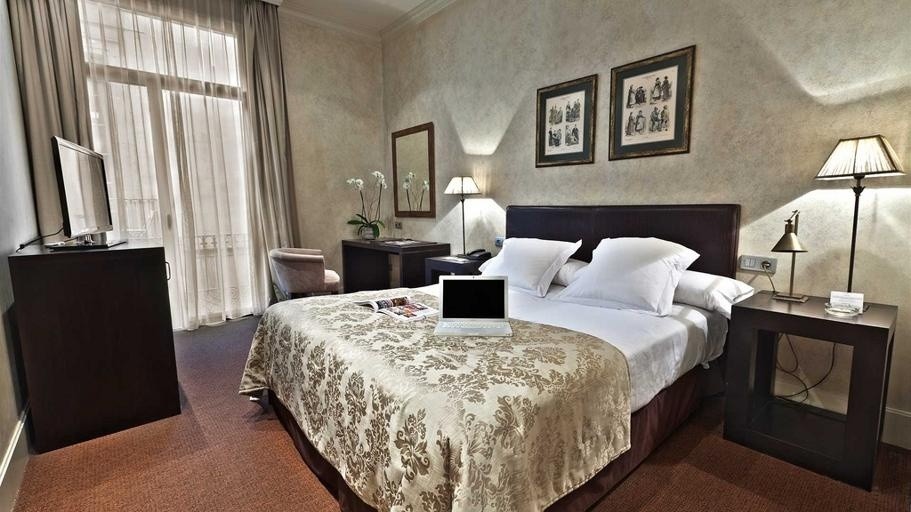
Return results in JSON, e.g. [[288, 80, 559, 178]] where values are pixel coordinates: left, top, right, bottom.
[[740, 256, 777, 274], [495, 237, 504, 246]]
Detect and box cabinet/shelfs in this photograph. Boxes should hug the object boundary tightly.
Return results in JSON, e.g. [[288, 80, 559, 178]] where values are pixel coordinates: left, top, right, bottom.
[[8, 238, 182, 454], [723, 290, 898, 492]]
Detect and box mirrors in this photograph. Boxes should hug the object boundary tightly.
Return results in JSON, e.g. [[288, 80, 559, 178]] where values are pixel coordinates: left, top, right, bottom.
[[392, 122, 435, 217]]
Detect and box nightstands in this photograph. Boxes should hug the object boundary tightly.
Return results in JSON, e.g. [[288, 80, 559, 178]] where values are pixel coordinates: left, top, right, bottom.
[[424, 257, 487, 286]]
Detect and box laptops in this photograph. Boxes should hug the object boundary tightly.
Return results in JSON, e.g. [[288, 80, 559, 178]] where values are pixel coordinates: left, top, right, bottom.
[[433, 275, 514, 337]]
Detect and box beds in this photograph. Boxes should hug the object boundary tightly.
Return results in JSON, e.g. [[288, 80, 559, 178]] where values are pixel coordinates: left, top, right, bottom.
[[238, 203, 755, 512]]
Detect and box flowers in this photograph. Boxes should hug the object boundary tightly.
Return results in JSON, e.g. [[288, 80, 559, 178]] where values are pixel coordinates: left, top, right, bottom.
[[402, 172, 429, 212], [346, 170, 388, 238]]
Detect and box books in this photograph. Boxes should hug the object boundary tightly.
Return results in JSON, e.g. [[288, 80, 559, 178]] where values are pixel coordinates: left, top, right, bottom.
[[353, 295, 440, 323]]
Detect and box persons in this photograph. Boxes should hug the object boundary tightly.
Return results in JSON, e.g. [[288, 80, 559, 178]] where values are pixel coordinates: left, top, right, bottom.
[[624, 76, 671, 135], [549, 98, 581, 146]]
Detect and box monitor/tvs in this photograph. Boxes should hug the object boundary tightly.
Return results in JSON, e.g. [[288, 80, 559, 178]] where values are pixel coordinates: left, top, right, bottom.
[[49, 135, 128, 249]]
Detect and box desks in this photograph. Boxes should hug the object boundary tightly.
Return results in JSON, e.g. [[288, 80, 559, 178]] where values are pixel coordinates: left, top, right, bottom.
[[342, 238, 450, 294]]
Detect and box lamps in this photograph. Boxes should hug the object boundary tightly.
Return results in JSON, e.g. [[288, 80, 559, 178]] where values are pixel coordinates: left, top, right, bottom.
[[813, 136, 906, 291], [769, 210, 808, 300], [443, 176, 482, 255]]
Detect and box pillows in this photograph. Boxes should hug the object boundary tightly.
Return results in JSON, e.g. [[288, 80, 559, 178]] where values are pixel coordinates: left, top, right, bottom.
[[554, 258, 754, 319], [550, 236, 701, 316], [482, 236, 582, 298]]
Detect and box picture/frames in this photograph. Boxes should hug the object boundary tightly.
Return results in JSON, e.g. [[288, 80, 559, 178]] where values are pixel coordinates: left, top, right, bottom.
[[608, 45, 695, 161], [535, 74, 597, 167]]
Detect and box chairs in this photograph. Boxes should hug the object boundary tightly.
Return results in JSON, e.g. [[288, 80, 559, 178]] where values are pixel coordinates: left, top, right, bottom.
[[269, 247, 340, 301]]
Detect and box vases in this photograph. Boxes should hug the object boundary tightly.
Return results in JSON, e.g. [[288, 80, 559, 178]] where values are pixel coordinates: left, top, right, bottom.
[[361, 227, 375, 240]]
